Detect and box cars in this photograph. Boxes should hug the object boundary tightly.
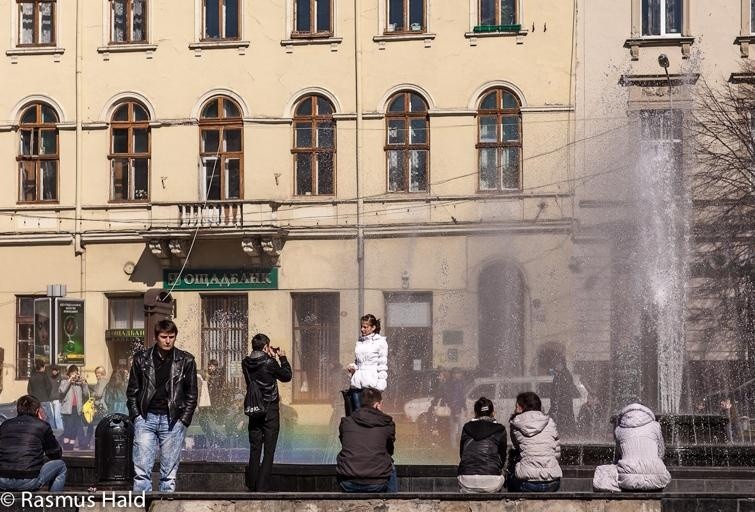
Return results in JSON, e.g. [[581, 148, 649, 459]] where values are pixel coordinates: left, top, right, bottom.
[[404, 374, 603, 447]]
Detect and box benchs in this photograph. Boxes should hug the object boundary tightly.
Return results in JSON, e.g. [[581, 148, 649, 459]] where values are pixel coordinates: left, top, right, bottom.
[[0, 490, 664, 512]]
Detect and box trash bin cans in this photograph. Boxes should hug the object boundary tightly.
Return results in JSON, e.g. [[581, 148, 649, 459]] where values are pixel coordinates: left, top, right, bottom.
[[95, 413, 134, 491]]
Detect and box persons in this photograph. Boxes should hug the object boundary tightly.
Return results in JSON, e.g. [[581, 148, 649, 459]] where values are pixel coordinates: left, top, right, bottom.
[[544, 359, 603, 442], [194, 356, 242, 449], [25, 360, 132, 450], [607, 403, 673, 494], [125, 319, 198, 493], [335, 313, 399, 493], [431, 363, 469, 448], [242, 333, 292, 491], [0, 394, 67, 494], [459, 391, 564, 493]]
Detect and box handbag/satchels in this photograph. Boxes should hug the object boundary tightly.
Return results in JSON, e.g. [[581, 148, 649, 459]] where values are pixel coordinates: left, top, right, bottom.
[[340, 373, 354, 416], [593, 464, 621, 493], [432, 398, 451, 417]]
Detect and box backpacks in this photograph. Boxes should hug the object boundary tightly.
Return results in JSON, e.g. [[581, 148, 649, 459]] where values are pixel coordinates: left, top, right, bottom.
[[82, 396, 96, 424]]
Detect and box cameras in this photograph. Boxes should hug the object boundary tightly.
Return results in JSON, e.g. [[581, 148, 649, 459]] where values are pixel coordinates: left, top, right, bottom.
[[272, 348, 280, 353], [76, 375, 80, 379]]
